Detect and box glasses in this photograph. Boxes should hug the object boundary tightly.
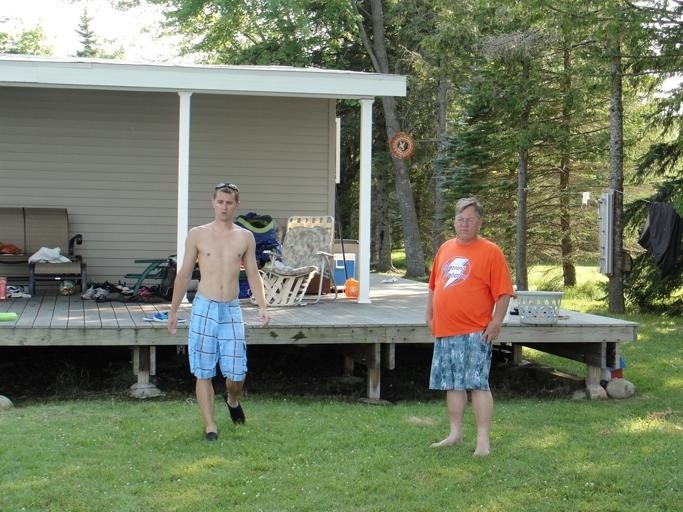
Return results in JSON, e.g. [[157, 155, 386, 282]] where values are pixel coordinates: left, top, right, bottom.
[[215, 182, 238, 191], [455, 216, 476, 224]]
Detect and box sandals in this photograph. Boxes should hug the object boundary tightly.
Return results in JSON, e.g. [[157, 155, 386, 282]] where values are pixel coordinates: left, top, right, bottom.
[[204, 430, 218, 441], [222, 390, 245, 424]]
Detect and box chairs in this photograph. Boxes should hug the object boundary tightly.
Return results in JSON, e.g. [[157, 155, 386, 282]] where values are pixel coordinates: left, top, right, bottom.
[[248, 215, 337, 307], [0, 207, 28, 301], [23, 207, 86, 297]]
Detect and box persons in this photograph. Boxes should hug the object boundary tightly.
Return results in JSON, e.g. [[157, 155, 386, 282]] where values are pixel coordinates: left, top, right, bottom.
[[167, 182, 270, 442], [423, 198, 515, 460]]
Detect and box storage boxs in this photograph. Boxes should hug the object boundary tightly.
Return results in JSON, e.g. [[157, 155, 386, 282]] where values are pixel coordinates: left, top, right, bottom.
[[333, 253, 355, 286]]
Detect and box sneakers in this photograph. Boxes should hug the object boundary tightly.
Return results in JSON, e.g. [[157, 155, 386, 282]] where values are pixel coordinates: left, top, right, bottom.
[[80, 285, 111, 300], [154, 311, 186, 322], [6, 286, 32, 299]]
